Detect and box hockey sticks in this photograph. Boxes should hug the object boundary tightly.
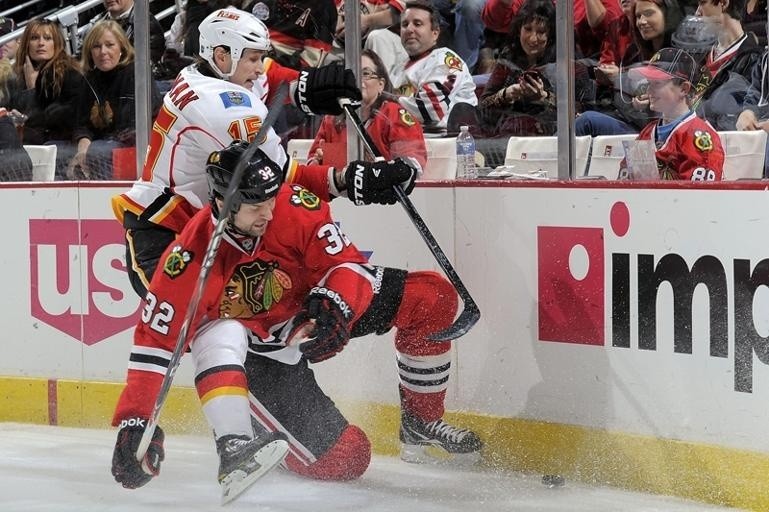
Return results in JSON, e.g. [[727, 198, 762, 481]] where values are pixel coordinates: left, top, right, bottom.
[[136, 78, 290, 462], [346, 105, 480, 342]]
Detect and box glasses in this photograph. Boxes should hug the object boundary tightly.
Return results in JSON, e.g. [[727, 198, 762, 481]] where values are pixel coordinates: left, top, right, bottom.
[[361, 67, 380, 80]]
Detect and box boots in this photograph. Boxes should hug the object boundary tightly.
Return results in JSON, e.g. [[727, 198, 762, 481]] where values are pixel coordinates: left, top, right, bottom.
[[398, 383, 482, 471], [211, 427, 290, 507]]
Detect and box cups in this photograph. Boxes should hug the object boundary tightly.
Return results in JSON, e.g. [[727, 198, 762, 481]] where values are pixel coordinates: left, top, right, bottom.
[[6, 111, 26, 150]]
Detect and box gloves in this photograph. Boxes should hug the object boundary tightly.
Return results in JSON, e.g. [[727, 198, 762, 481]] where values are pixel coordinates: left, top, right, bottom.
[[294, 63, 363, 117], [111, 415, 166, 489], [291, 285, 355, 365], [342, 157, 415, 207]]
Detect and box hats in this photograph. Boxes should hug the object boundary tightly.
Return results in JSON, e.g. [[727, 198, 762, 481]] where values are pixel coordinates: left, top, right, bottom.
[[630, 47, 696, 85]]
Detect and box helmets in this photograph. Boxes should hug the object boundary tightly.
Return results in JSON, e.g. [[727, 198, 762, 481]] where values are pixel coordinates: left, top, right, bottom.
[[204, 138, 283, 212], [196, 7, 271, 78]]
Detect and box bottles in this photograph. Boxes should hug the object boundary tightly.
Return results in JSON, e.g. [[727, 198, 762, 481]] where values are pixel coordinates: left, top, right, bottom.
[[456, 126, 477, 179]]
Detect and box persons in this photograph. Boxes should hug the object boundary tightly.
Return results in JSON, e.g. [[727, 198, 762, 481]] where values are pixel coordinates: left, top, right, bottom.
[[109, 139, 482, 484], [110, 6, 418, 482], [0, 1, 769, 183]]
